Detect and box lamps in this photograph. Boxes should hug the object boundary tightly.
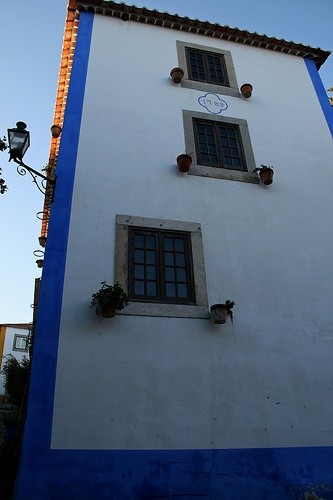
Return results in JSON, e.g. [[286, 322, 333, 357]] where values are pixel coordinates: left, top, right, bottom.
[[7, 121, 59, 204]]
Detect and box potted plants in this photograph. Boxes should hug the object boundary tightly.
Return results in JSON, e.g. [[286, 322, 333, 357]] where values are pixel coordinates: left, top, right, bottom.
[[170, 67, 183, 83], [50, 124, 62, 138], [89, 279, 129, 317], [177, 154, 193, 173], [253, 164, 274, 185], [240, 84, 253, 98], [210, 299, 236, 324]]
